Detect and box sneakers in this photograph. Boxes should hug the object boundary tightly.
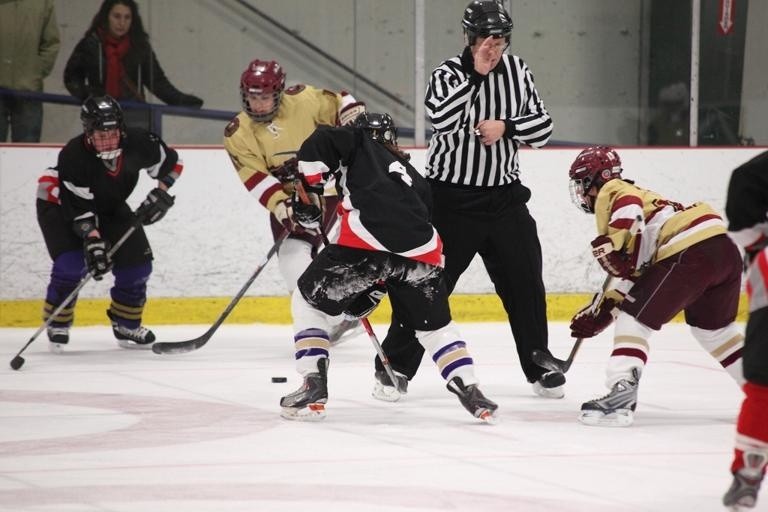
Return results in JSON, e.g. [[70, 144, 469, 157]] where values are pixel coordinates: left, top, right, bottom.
[[724, 477, 759, 505]]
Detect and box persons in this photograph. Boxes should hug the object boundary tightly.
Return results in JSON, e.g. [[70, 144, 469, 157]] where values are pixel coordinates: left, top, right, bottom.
[[37, 92, 184, 345], [279, 111, 499, 419], [569, 145, 747, 416], [224, 60, 368, 382], [373, 0, 568, 393], [63, 0, 204, 105], [1, 1, 58, 144], [721, 149, 768, 508]]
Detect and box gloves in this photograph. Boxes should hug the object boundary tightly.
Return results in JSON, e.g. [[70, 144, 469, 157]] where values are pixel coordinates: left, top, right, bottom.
[[71, 211, 114, 280], [590, 233, 637, 280], [343, 281, 388, 321], [274, 194, 301, 233], [570, 294, 620, 338], [292, 187, 325, 229], [134, 189, 174, 224]]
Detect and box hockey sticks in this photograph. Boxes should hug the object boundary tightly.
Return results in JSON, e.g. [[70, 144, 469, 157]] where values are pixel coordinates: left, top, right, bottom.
[[151, 228, 296, 355], [10, 220, 147, 370], [533, 270, 613, 373], [296, 181, 407, 395]]
